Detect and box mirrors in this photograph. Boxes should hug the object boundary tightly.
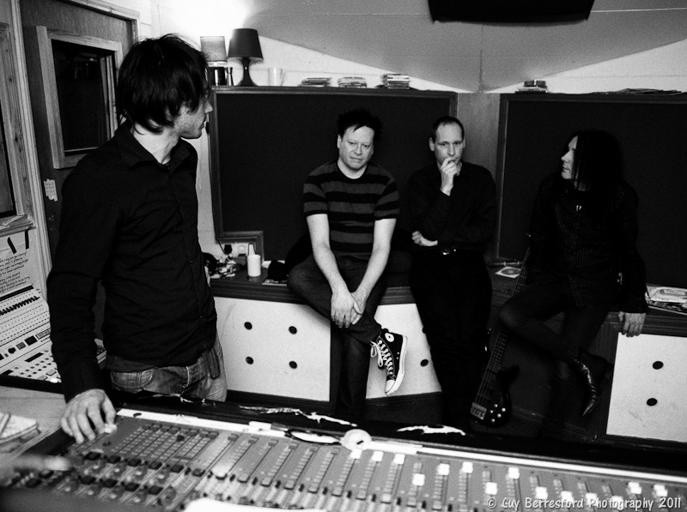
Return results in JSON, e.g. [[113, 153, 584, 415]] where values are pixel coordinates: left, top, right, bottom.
[[35, 25, 127, 170]]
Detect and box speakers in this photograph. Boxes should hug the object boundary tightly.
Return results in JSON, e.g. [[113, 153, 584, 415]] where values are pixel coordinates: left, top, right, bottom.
[[428, 0, 593, 26]]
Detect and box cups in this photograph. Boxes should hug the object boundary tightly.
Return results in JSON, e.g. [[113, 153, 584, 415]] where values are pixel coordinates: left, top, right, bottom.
[[269, 67, 283, 86], [246, 254, 262, 278]]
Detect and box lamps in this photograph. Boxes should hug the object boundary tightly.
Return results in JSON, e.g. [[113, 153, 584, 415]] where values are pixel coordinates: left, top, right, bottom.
[[227, 28, 264, 87]]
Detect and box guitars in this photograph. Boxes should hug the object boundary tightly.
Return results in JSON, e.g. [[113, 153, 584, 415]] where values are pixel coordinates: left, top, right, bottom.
[[470, 236, 540, 427]]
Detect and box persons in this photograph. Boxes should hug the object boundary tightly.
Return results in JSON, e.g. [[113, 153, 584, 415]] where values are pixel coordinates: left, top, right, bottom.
[[496, 127, 651, 458], [41, 31, 231, 445], [400, 113, 501, 415], [279, 108, 410, 422]]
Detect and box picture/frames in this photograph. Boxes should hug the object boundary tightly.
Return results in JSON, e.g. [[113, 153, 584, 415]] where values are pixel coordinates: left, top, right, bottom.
[[0, 23, 39, 238]]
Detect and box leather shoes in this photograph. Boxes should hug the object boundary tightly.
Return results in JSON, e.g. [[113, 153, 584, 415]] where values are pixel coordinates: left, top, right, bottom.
[[575, 354, 606, 418]]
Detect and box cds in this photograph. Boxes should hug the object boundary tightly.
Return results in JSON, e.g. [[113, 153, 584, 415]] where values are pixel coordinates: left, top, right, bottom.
[[501, 269, 520, 275]]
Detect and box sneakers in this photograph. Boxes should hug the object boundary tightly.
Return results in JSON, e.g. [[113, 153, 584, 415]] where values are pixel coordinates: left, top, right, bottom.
[[369, 328, 407, 395]]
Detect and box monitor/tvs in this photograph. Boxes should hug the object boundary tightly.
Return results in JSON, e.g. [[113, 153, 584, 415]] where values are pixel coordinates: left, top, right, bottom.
[[496, 93, 687, 264], [210, 86, 458, 268]]
